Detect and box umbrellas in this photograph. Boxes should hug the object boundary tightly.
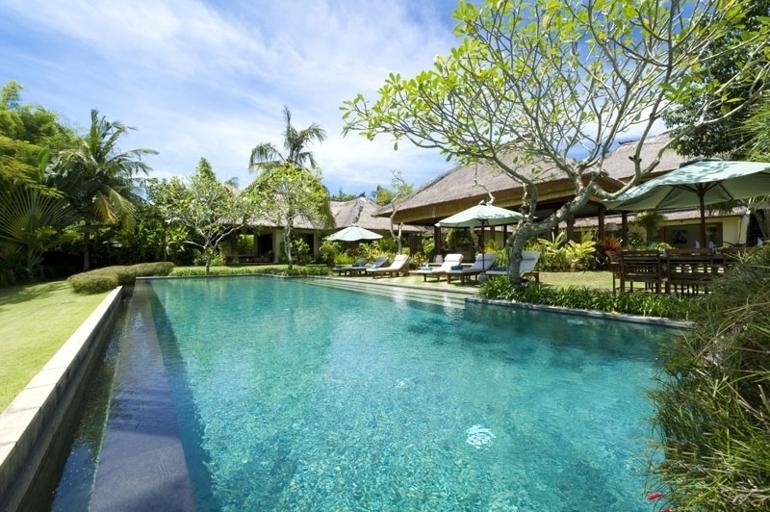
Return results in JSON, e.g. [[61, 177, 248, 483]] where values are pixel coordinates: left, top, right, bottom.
[[323, 224, 386, 265], [430, 198, 541, 271], [601, 153, 770, 291]]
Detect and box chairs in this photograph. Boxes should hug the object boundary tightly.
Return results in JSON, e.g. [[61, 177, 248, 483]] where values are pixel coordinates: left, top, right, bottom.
[[331, 250, 543, 286], [605, 246, 753, 295]]
